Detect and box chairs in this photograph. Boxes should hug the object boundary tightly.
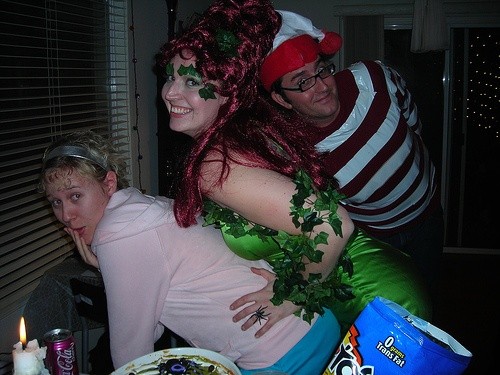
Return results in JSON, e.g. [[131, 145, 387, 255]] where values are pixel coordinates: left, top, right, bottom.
[[69, 278, 178, 375]]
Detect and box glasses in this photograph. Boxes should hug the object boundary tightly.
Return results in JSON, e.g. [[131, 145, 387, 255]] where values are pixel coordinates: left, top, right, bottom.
[[275, 59, 336, 94]]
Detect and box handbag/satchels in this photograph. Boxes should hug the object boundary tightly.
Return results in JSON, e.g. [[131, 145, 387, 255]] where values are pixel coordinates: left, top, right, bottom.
[[322, 297, 473, 375]]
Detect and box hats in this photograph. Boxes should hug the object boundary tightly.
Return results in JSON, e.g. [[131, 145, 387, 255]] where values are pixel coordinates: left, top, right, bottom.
[[258, 9, 343, 92]]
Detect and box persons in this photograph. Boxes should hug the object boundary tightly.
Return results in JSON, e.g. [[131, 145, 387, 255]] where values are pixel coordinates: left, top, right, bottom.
[[36, 129, 340, 375], [257, 11, 445, 296], [156, 1, 433, 338]]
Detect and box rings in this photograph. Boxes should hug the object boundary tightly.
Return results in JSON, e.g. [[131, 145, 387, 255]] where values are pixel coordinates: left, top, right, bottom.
[[249, 304, 271, 324]]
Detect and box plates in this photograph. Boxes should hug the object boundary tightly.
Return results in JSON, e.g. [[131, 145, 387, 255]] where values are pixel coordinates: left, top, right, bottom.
[[110, 347, 242, 375]]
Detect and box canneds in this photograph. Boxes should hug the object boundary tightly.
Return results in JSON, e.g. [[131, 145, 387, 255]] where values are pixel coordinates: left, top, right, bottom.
[[41, 328, 78, 375]]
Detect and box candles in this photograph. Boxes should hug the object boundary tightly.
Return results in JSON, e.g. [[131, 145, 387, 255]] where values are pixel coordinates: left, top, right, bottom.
[[12, 316, 50, 375]]
